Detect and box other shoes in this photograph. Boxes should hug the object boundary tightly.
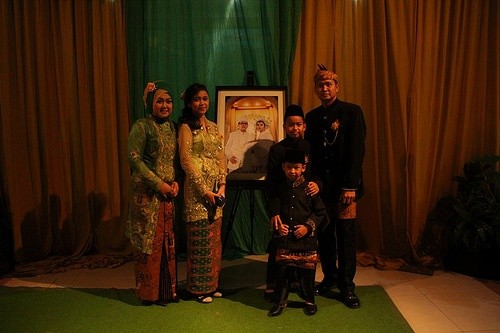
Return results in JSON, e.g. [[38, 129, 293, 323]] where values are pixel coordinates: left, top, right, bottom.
[[142, 300, 152, 306], [159, 301, 167, 306], [211, 291, 222, 297], [264, 291, 274, 303], [196, 294, 213, 302]]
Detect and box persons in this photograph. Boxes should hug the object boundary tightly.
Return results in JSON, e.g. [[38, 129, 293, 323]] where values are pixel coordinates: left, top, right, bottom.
[[226, 117, 251, 170], [127, 83, 183, 308], [255, 116, 274, 143], [302, 65, 366, 310], [268, 146, 322, 316], [175, 83, 228, 304], [264, 102, 325, 297]]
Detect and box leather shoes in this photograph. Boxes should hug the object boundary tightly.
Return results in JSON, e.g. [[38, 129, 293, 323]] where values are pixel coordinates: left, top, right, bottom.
[[313, 278, 336, 295], [269, 303, 286, 316], [305, 301, 317, 314], [341, 291, 359, 309]]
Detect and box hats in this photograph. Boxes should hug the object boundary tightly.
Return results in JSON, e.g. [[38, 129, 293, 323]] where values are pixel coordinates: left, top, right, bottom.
[[284, 104, 304, 121], [283, 147, 305, 164], [314, 64, 338, 83]]
